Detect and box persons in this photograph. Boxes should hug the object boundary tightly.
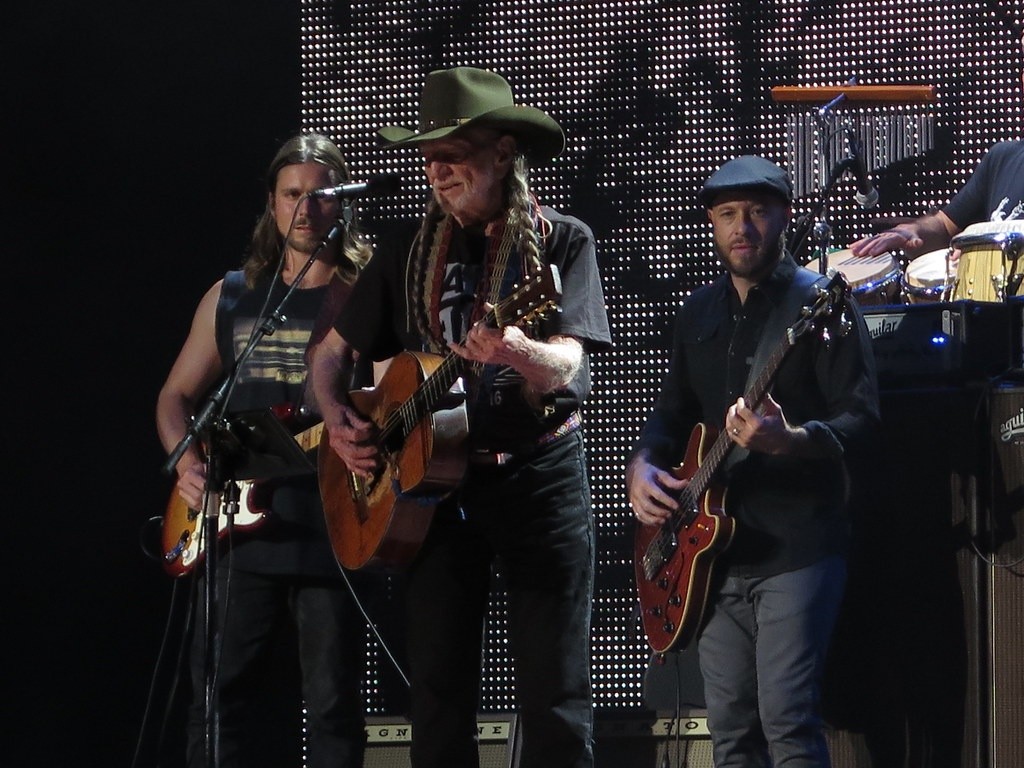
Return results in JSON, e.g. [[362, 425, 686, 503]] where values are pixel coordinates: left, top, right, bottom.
[[624, 154, 883, 768], [154, 133, 406, 768], [308, 66, 615, 768], [848, 139, 1024, 258]]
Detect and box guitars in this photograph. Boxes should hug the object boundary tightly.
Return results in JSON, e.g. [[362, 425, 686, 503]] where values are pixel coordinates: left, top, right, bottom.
[[632, 274, 852, 654], [316, 259, 564, 575], [162, 226, 386, 581]]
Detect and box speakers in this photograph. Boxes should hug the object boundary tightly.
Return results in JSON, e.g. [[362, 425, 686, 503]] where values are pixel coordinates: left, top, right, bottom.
[[362, 712, 522, 768], [650, 383, 1024, 768]]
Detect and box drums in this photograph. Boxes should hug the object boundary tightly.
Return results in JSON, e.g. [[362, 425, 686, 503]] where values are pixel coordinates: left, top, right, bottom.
[[950, 221, 1023, 304], [901, 246, 961, 303], [802, 248, 903, 305]]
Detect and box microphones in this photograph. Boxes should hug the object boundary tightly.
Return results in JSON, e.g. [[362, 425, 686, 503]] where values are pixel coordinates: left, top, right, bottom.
[[304, 172, 400, 199], [845, 128, 878, 209]]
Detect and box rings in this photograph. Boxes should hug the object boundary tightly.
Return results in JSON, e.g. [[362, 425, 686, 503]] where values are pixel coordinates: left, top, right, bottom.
[[731, 427, 741, 437], [632, 512, 639, 520]]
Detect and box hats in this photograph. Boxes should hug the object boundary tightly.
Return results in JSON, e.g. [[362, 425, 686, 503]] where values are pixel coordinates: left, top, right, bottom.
[[379, 67, 564, 169], [698, 156, 792, 208]]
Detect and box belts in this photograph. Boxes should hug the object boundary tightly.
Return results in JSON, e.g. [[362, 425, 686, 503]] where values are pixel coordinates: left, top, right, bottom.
[[473, 409, 584, 466]]
